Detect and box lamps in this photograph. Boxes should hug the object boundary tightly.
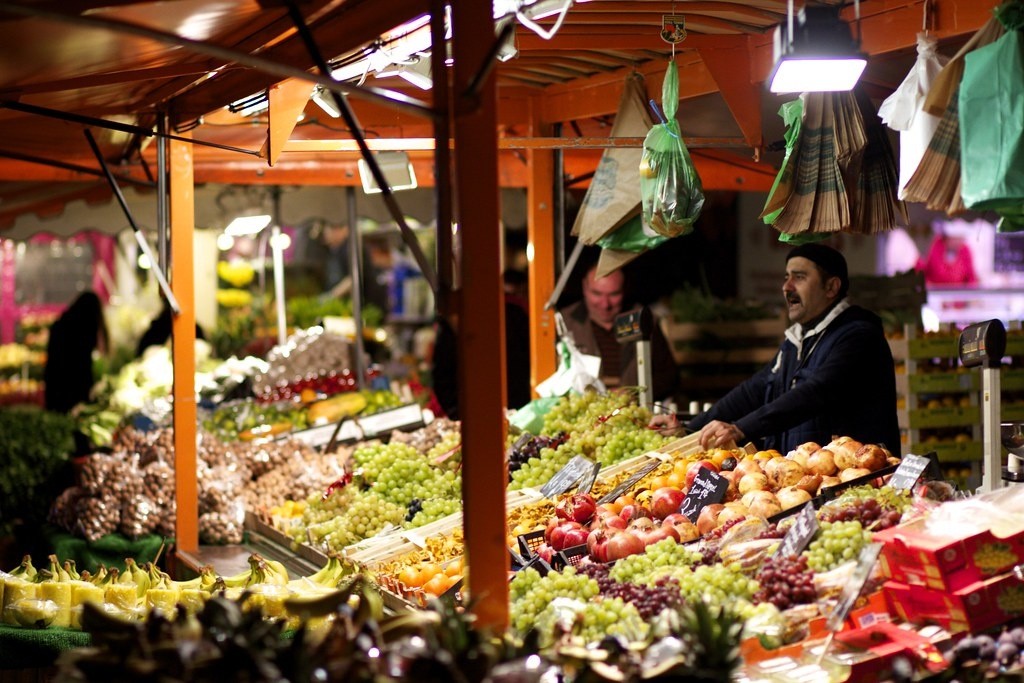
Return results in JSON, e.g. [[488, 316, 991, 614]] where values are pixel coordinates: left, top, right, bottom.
[[357, 149, 418, 194], [314, 89, 341, 118], [397, 51, 433, 92], [763, 0, 869, 93], [222, 205, 271, 237]]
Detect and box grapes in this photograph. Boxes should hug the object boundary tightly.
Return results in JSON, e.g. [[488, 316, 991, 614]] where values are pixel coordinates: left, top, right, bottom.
[[509, 482, 910, 645], [279, 386, 680, 553]]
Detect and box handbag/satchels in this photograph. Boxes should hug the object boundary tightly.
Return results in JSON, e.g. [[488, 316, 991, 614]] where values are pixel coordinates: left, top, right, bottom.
[[639, 60, 705, 238]]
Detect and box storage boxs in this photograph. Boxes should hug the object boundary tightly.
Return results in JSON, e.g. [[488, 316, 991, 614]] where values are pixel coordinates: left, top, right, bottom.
[[739, 481, 1024, 683]]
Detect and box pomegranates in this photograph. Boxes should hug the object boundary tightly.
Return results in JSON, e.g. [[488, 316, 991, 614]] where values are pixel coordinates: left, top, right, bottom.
[[541, 435, 901, 560]]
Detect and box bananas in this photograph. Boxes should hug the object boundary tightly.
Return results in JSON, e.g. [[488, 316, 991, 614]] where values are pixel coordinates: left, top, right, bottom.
[[6, 554, 494, 683]]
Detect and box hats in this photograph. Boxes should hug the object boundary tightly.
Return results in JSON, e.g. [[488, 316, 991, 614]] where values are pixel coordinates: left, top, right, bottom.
[[787, 243, 848, 300]]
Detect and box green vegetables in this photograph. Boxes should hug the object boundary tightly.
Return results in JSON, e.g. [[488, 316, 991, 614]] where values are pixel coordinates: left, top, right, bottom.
[[0, 402, 92, 522]]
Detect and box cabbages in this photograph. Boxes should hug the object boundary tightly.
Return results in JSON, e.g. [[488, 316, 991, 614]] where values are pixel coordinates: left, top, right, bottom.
[[88, 342, 173, 444]]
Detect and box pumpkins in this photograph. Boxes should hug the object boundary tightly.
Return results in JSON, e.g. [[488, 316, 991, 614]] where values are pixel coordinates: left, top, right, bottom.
[[307, 392, 365, 427], [238, 423, 292, 440]]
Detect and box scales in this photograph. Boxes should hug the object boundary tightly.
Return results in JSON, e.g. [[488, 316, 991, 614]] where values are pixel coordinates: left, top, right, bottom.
[[955, 318, 1024, 496], [615, 306, 697, 425]]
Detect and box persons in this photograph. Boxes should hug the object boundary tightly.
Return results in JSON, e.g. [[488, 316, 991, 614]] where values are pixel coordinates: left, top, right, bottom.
[[134, 298, 205, 357], [431, 300, 530, 422], [555, 261, 688, 410], [293, 217, 420, 316], [227, 216, 295, 265], [41, 288, 109, 414], [649, 243, 901, 461]]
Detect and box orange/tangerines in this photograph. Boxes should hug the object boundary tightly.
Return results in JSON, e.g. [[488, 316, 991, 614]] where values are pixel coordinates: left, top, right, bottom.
[[205, 391, 402, 443]]
